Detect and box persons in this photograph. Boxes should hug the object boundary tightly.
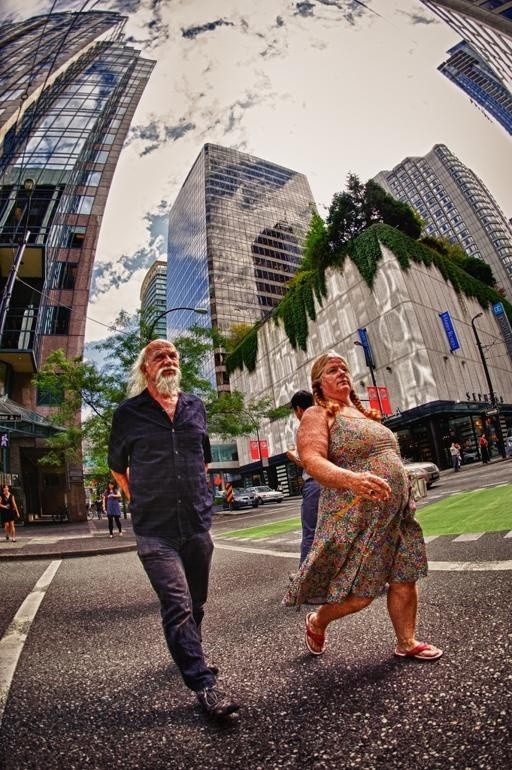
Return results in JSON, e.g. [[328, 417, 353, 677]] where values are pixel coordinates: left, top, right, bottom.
[[455, 442, 462, 469], [284, 389, 322, 582], [95, 496, 103, 520], [0, 484, 21, 542], [101, 479, 123, 538], [449, 442, 460, 472], [107, 337, 241, 718], [479, 437, 490, 461], [277, 482, 283, 492], [479, 433, 490, 463], [295, 350, 444, 662]]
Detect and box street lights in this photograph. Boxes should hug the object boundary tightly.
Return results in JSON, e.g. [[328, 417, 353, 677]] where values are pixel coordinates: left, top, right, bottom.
[[148, 307, 208, 341], [472, 311, 507, 458], [353, 340, 386, 418]]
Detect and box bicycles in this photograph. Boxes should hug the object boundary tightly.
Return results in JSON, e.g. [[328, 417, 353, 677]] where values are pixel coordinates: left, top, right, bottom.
[[53, 502, 94, 523]]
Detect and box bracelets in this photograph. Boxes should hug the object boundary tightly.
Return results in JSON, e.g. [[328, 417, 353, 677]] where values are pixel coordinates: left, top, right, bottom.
[[103, 510, 106, 512], [295, 456, 301, 465]]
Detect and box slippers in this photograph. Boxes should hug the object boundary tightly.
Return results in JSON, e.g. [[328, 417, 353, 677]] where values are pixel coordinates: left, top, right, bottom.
[[394, 642, 443, 660], [305, 612, 326, 655]]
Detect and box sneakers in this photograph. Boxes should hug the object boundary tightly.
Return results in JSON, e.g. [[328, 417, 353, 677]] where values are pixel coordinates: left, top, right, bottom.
[[109, 535, 113, 538], [119, 532, 123, 536], [195, 666, 238, 719]]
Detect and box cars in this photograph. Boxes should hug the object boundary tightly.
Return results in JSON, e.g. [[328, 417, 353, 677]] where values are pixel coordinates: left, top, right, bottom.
[[216, 485, 285, 508], [397, 458, 441, 490]]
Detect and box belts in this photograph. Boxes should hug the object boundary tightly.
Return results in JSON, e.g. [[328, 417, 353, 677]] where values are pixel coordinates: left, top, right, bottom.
[[305, 478, 313, 482]]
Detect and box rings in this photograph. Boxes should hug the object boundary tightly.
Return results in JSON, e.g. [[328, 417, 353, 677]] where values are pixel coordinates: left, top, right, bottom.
[[369, 489, 376, 496]]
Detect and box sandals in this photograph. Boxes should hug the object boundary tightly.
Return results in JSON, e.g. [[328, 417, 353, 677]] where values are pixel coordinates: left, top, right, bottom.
[[5, 536, 16, 542]]
